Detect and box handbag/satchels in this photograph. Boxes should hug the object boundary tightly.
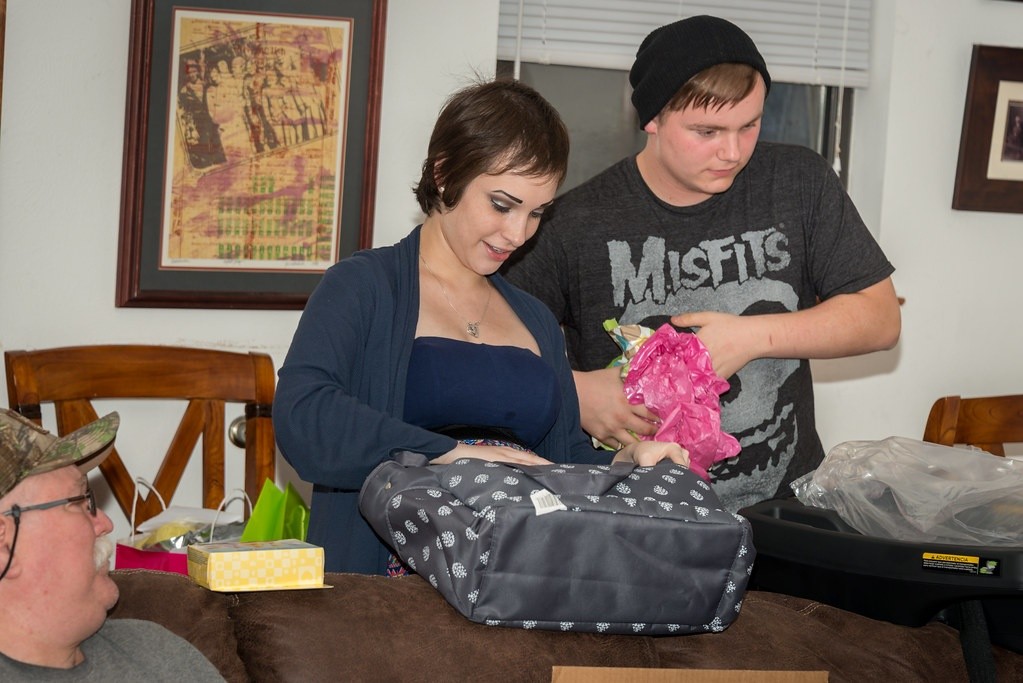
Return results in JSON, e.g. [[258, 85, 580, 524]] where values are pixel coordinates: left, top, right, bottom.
[[117, 476, 252, 575], [240, 479, 310, 545], [357, 449, 756, 634]]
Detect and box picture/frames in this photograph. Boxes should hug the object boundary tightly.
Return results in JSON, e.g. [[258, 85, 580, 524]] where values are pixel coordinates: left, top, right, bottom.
[[112, 0, 387, 313], [946, 45, 1023, 215]]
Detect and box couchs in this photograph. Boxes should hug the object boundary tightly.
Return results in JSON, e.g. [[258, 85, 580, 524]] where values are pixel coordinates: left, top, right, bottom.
[[108, 566, 1021, 682]]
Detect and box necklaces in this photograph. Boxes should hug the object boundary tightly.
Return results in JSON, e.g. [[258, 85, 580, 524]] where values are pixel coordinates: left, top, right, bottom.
[[418, 253, 491, 339]]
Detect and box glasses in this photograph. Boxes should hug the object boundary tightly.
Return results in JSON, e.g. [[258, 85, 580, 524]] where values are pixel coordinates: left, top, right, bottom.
[[2, 488, 98, 518]]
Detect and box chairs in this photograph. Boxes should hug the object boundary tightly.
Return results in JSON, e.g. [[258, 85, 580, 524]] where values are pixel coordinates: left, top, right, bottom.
[[6, 344, 278, 556]]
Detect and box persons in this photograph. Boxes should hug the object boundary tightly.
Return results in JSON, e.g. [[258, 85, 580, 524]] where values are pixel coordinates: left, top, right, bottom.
[[500, 15, 902, 516], [177, 35, 345, 167], [0, 409, 230, 683], [269, 76, 691, 576]]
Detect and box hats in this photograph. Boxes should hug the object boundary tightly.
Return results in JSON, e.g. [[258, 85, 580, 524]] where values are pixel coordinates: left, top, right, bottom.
[[0, 405, 121, 500], [630, 15, 772, 129]]
